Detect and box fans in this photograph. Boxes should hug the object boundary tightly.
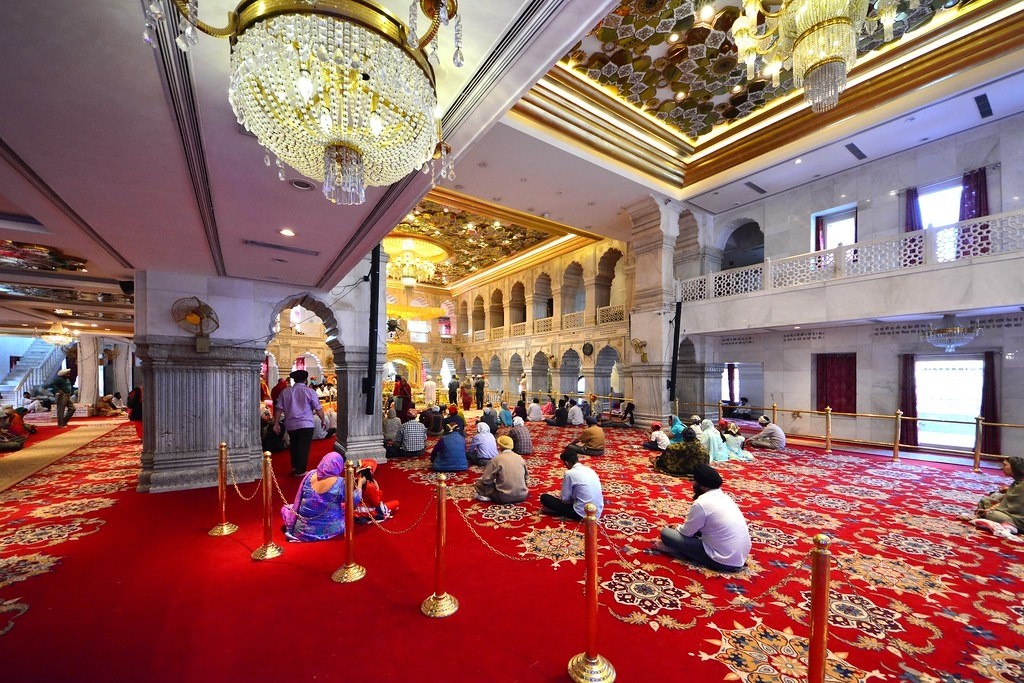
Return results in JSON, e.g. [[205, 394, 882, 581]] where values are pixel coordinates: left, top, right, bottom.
[[453, 345, 464, 357], [544, 354, 557, 368], [170, 295, 220, 353], [385, 313, 407, 340], [98, 349, 120, 366], [630, 338, 648, 362]]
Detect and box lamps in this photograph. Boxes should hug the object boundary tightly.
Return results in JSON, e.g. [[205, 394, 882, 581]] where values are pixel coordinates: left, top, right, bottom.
[[139, 0, 466, 206], [33, 322, 79, 346], [730, 0, 923, 114], [380, 230, 454, 286]]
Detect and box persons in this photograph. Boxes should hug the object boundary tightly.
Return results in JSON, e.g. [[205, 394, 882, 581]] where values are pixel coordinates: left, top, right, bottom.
[[383, 397, 532, 502], [393, 374, 411, 424], [976, 456, 1024, 534], [643, 415, 754, 480], [746, 415, 785, 448], [53, 369, 76, 428], [651, 465, 751, 574], [597, 403, 635, 428], [0, 405, 30, 439], [733, 397, 751, 419], [260, 370, 337, 476], [518, 373, 527, 401], [540, 450, 604, 521], [475, 394, 603, 427], [127, 387, 143, 441], [563, 417, 605, 455], [448, 373, 491, 411], [23, 383, 54, 412], [101, 392, 128, 416], [423, 375, 437, 407], [281, 452, 399, 541]]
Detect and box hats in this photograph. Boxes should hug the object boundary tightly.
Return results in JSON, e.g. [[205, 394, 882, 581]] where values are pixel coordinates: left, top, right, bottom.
[[741, 396, 748, 402], [289, 370, 309, 381], [448, 406, 458, 414], [407, 408, 417, 417], [586, 416, 597, 424], [497, 435, 514, 449], [444, 422, 460, 433], [260, 373, 264, 376], [758, 415, 771, 423], [651, 422, 662, 431], [691, 414, 701, 421], [432, 406, 440, 412], [719, 420, 728, 428], [476, 375, 481, 377], [426, 375, 432, 379], [693, 464, 723, 489], [682, 427, 696, 439], [452, 375, 455, 378]]
